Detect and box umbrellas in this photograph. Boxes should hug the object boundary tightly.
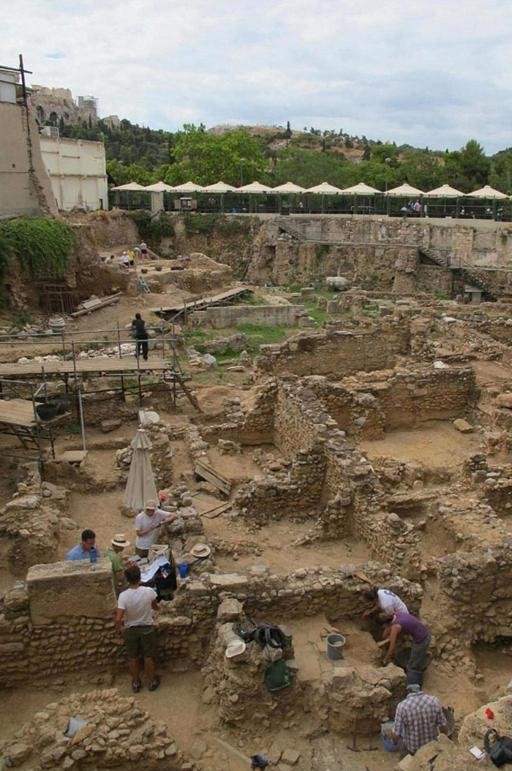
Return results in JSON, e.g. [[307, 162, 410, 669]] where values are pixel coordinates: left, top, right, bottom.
[[123, 429, 161, 509]]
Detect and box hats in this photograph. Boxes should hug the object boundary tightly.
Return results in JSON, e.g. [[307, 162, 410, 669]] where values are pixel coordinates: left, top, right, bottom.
[[142, 500, 157, 510], [190, 542, 210, 557], [223, 640, 247, 658], [111, 530, 131, 549]]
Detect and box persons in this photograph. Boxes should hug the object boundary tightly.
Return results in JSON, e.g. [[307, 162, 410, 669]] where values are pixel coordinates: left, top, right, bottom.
[[140, 239, 151, 264], [115, 565, 162, 693], [139, 276, 145, 297], [385, 670, 449, 763], [132, 314, 148, 361], [131, 541, 177, 603], [104, 534, 136, 599], [122, 251, 129, 269], [65, 530, 101, 564], [103, 255, 114, 265], [132, 500, 178, 559], [360, 589, 409, 648], [376, 612, 431, 670], [128, 248, 135, 266]]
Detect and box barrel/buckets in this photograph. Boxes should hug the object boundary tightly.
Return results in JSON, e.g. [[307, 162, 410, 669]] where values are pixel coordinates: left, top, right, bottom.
[[326, 634, 346, 660]]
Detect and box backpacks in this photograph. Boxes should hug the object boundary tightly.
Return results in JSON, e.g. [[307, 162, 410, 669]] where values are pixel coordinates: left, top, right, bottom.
[[255, 623, 284, 648]]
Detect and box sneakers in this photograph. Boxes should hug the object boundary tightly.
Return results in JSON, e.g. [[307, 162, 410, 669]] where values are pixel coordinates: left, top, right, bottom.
[[131, 675, 142, 693], [148, 674, 161, 692]]
